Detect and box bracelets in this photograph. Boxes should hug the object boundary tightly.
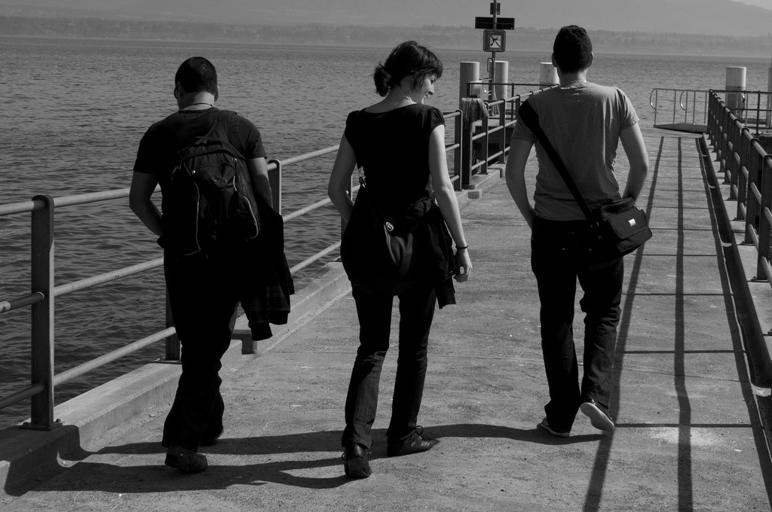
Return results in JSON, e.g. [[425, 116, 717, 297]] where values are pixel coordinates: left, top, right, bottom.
[[456, 246, 468, 249]]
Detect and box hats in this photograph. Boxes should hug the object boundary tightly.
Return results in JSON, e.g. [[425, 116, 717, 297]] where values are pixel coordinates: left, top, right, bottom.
[[554, 26, 592, 65]]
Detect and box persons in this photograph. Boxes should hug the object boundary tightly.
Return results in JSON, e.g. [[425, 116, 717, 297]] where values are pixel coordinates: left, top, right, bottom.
[[128, 54, 273, 474], [504, 24, 649, 440], [328, 39, 472, 480]]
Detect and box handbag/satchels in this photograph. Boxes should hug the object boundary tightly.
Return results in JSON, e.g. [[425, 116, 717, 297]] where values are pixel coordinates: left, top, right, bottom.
[[588, 196, 652, 255]]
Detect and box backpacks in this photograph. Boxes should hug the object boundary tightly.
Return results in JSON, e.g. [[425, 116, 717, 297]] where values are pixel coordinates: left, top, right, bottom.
[[171, 110, 259, 256]]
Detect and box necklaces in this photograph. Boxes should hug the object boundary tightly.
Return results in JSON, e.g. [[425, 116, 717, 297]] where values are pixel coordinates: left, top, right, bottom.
[[186, 102, 214, 107]]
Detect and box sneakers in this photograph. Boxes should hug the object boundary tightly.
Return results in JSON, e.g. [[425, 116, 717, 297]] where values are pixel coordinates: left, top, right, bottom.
[[164, 450, 209, 474], [540, 417, 569, 437], [580, 398, 615, 432], [198, 425, 223, 447], [344, 443, 372, 478], [389, 433, 440, 456]]
